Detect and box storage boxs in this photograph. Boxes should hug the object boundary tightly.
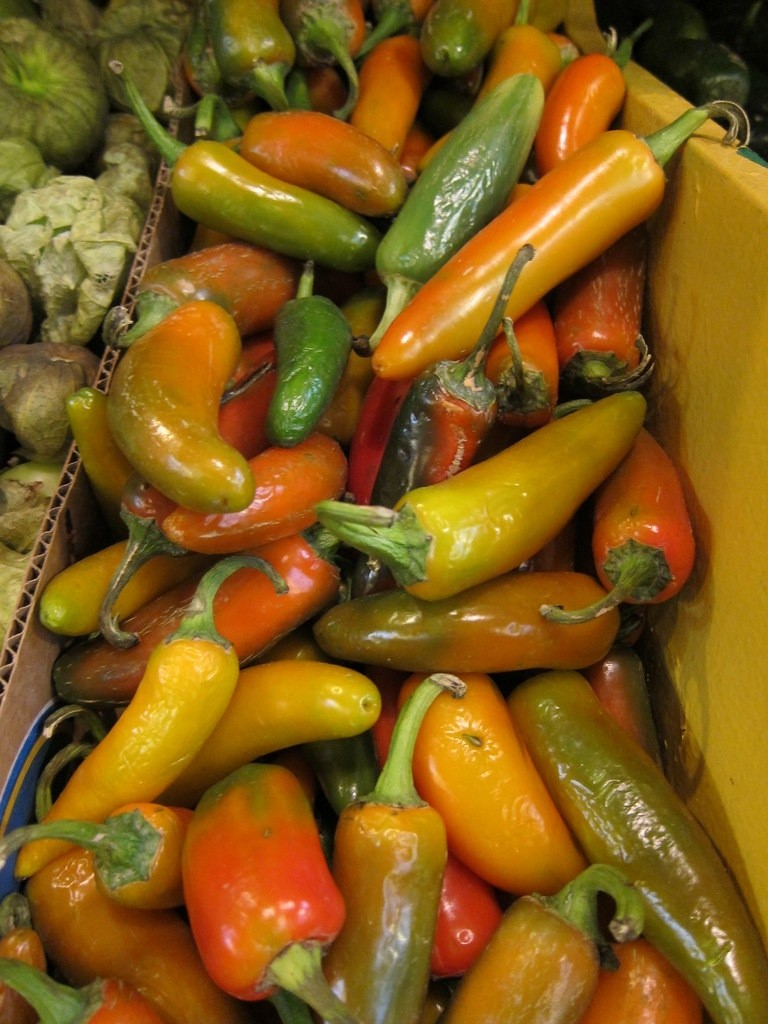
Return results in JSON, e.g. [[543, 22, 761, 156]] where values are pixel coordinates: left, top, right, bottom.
[[0, 0, 768, 1024]]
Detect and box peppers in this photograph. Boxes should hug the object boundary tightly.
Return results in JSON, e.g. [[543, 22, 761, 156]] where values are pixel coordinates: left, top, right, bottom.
[[0, 0, 768, 1024]]
